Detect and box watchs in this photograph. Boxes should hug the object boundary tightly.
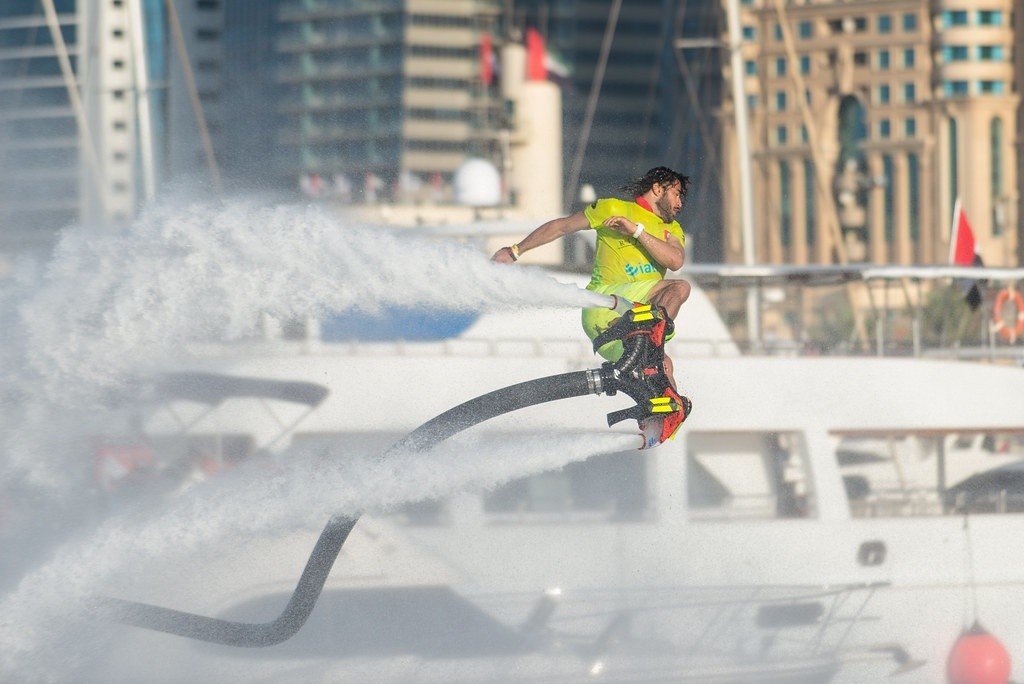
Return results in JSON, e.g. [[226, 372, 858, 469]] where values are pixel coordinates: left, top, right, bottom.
[[633, 223, 645, 238]]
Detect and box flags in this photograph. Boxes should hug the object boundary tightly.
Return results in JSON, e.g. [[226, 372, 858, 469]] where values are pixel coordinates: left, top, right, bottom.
[[480, 35, 491, 84], [527, 27, 549, 82], [952, 209, 975, 267]]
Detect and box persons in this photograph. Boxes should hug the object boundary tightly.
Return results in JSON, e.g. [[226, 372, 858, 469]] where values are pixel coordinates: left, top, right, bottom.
[[490, 167, 692, 429]]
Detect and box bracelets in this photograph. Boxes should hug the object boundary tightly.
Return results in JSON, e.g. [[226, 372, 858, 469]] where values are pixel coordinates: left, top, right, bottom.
[[506, 244, 520, 262]]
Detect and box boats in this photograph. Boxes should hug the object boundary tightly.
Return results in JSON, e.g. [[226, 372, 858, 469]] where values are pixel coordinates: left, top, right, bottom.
[[0, 0, 1024, 684]]
